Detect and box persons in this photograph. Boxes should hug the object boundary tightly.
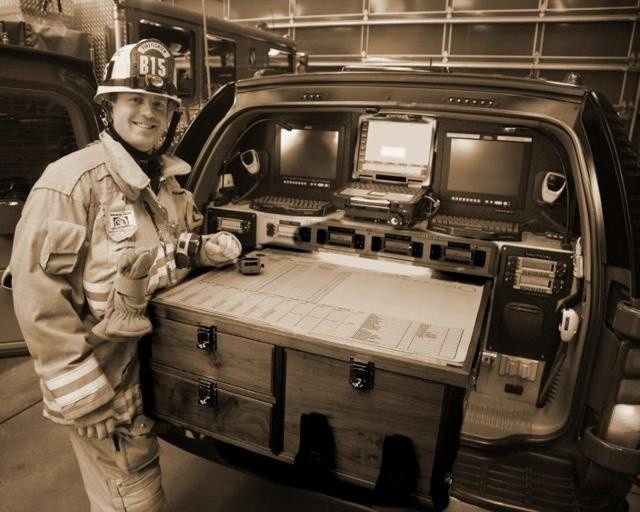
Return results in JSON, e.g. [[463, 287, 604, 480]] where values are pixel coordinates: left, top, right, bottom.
[[10, 35, 244, 512]]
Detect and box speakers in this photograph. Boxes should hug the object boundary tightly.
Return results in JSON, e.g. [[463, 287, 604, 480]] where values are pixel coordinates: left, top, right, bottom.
[[239, 148, 261, 176], [539, 172, 567, 205]]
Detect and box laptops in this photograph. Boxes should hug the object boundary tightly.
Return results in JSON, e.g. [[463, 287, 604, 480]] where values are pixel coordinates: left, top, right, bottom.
[[339, 116, 438, 210]]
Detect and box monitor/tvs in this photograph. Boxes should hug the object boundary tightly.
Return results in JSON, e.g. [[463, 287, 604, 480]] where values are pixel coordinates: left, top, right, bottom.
[[271, 123, 346, 191], [438, 132, 534, 211]]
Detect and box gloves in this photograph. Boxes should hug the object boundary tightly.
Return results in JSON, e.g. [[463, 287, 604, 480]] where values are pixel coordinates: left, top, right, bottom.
[[90, 243, 158, 343]]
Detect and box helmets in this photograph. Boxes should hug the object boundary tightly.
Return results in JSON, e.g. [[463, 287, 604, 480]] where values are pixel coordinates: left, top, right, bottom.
[[91, 38, 182, 112]]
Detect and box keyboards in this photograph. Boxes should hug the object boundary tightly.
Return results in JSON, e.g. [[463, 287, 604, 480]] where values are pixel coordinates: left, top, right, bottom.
[[427, 213, 523, 242], [249, 194, 339, 218]]
